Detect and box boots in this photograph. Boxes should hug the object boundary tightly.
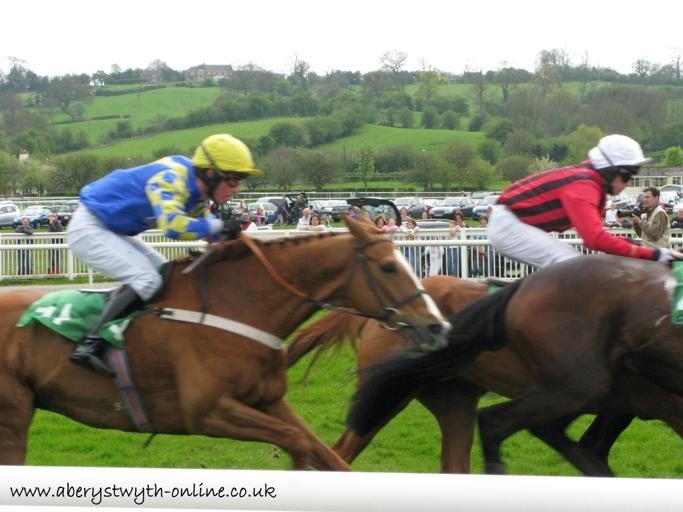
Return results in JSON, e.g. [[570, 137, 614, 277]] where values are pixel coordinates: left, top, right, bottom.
[[66, 282, 145, 380]]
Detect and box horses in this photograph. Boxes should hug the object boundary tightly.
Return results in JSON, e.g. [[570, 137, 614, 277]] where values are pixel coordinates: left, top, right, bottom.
[[0, 210, 452, 471], [336, 254, 683, 477], [286, 275, 516, 474]]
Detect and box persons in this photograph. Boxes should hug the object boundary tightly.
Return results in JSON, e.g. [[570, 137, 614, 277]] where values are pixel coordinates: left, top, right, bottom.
[[14, 218, 33, 275], [47, 212, 64, 275], [487, 134, 683, 268], [65, 133, 261, 380], [239, 191, 504, 280], [606, 187, 683, 250]]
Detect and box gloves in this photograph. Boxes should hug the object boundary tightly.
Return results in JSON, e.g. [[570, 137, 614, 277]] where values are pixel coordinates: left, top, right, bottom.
[[222, 218, 249, 235], [658, 247, 683, 265]]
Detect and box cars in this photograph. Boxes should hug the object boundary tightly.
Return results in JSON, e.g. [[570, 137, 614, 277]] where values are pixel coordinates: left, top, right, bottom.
[[209, 191, 502, 224], [603, 182, 683, 227], [0, 203, 77, 230]]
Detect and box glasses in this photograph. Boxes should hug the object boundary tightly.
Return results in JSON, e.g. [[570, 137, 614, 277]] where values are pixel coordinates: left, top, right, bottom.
[[618, 171, 632, 183], [224, 175, 244, 188]]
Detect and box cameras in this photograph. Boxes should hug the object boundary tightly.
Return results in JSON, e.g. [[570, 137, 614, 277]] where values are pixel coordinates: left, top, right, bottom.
[[617, 206, 641, 217]]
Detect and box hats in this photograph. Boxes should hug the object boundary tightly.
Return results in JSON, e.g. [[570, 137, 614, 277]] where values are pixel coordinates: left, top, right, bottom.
[[190, 130, 263, 178], [587, 132, 657, 173]]
